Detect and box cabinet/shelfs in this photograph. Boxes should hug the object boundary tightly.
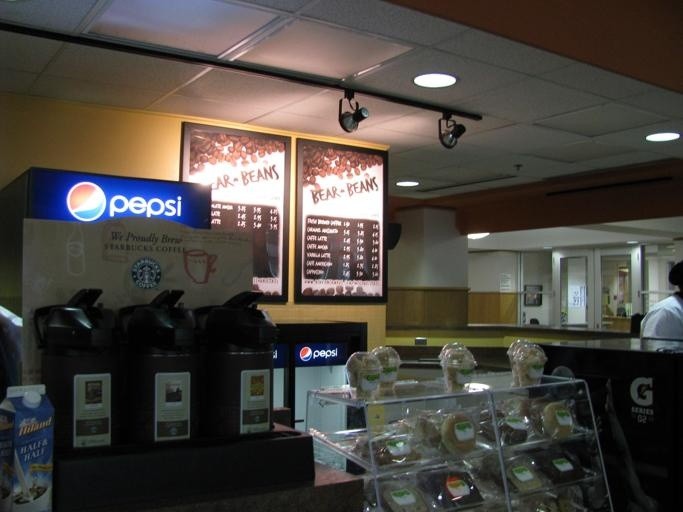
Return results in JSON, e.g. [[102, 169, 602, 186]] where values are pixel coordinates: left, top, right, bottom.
[[305, 369, 613, 508]]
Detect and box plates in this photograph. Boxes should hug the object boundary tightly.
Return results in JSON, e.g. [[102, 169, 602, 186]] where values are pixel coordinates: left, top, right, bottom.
[[361, 459, 501, 512], [463, 444, 600, 501], [328, 434, 495, 472], [463, 406, 596, 453], [503, 493, 586, 512], [444, 502, 524, 512]]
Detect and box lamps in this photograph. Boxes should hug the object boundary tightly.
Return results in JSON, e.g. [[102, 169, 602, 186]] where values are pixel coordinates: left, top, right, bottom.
[[338, 92, 370, 136], [438, 112, 467, 148]]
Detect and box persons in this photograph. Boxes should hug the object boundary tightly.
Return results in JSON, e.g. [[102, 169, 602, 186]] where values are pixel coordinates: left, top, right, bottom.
[[638, 261, 683, 341]]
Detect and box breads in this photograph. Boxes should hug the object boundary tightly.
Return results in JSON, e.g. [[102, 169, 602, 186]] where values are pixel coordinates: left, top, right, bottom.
[[371, 396, 611, 512]]
